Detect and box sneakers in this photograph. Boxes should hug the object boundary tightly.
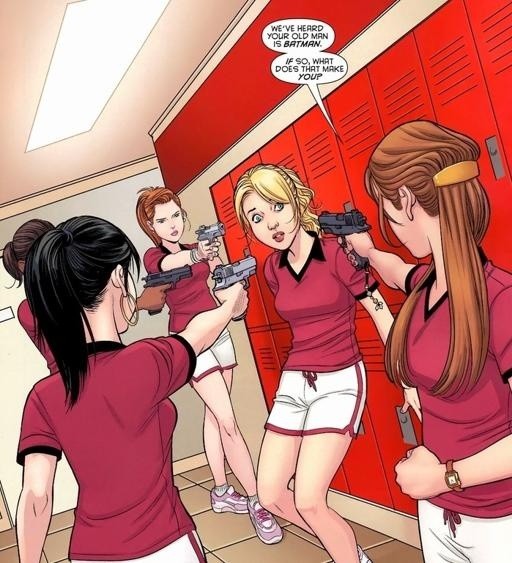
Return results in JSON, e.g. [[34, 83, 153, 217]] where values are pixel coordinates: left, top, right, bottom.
[[357, 544, 370, 563], [248, 499, 283, 545], [210, 485, 249, 513]]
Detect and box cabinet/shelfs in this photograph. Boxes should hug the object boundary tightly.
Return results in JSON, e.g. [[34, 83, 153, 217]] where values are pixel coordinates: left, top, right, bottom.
[[206, 0, 511, 519]]
